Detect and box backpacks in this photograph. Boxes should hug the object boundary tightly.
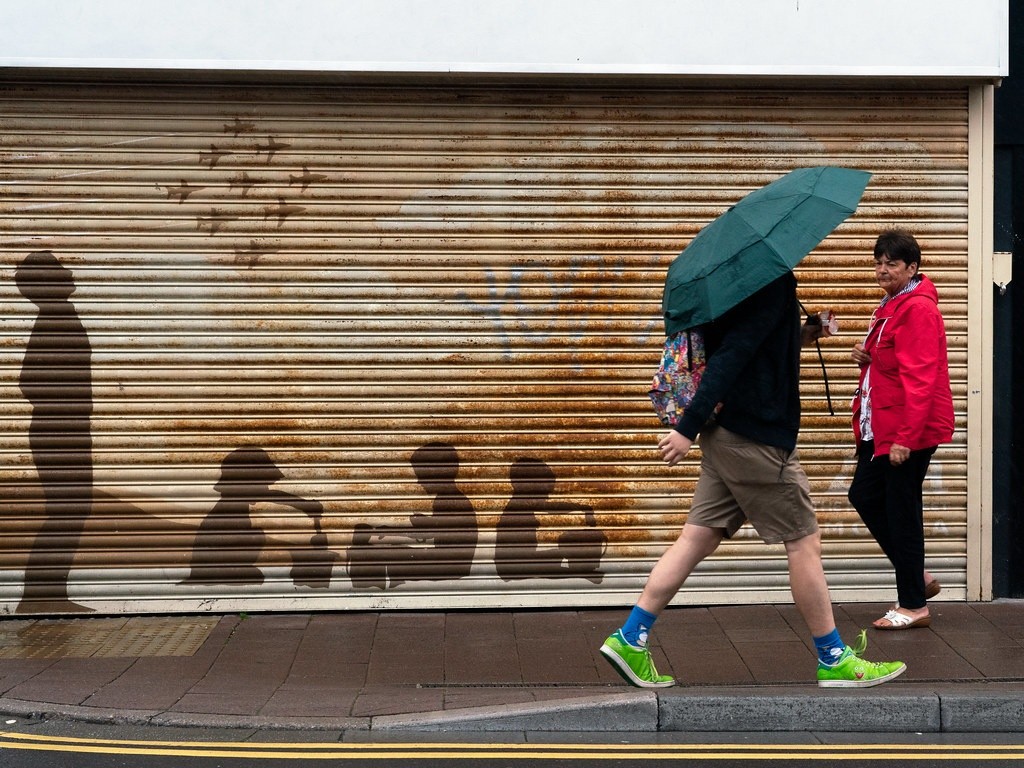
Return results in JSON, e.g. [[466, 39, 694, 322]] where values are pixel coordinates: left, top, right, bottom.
[[647, 331, 721, 426]]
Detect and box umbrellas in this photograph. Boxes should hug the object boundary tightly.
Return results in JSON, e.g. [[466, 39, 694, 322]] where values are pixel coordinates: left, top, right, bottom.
[[660, 165, 873, 336]]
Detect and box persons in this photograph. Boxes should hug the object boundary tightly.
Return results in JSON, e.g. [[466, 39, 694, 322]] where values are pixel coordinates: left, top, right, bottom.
[[848, 227, 954, 631], [597, 271, 908, 689]]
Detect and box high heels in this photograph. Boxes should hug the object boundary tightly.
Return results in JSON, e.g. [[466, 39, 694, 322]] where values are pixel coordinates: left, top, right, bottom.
[[890, 580, 941, 613], [878, 610, 931, 630]]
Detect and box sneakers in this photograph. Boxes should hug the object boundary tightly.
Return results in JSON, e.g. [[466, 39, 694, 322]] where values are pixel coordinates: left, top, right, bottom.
[[815, 629, 906, 690], [600, 627, 675, 688]]
